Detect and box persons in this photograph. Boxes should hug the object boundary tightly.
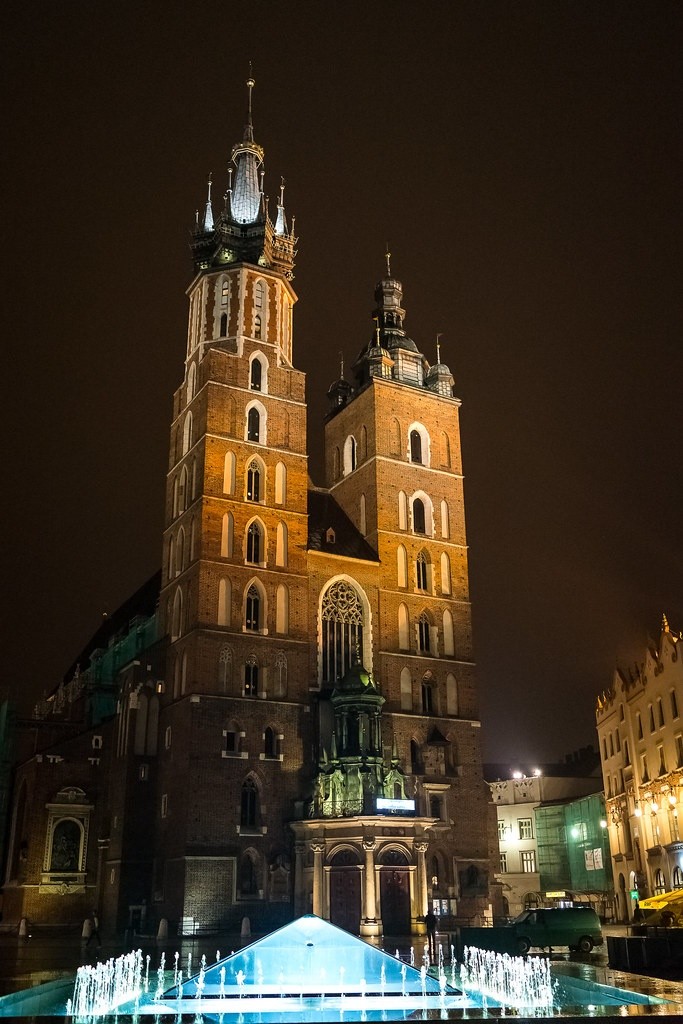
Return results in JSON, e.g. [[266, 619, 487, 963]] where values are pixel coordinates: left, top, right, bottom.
[[425, 910, 437, 944], [81, 911, 102, 948], [634, 904, 644, 923]]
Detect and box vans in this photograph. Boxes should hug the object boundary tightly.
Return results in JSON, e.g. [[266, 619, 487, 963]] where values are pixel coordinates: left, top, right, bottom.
[[507, 907, 604, 954]]
